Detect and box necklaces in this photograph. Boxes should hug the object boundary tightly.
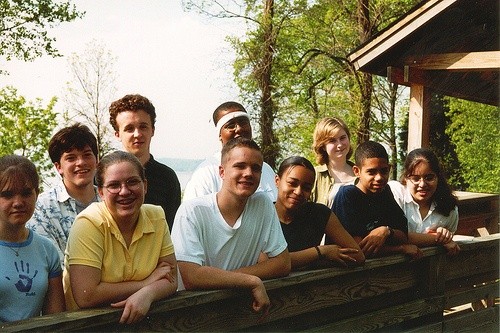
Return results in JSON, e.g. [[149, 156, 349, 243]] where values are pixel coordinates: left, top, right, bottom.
[[0, 229, 26, 256]]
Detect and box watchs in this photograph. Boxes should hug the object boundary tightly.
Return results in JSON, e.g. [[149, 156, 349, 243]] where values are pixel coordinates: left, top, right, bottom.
[[387, 225, 395, 243]]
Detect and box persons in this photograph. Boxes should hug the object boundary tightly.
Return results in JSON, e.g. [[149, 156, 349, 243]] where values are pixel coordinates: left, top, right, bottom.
[[259, 156, 366, 271], [25, 122, 103, 275], [0, 154, 66, 323], [109, 95, 182, 236], [324, 141, 423, 264], [183, 101, 278, 207], [62, 151, 178, 333], [388, 148, 461, 326], [170, 139, 291, 325], [309, 116, 354, 207]]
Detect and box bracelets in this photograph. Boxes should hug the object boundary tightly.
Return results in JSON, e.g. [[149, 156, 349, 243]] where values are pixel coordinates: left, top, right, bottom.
[[314, 246, 321, 258]]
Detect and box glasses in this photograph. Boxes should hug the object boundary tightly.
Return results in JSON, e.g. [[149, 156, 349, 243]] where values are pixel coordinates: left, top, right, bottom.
[[405, 173, 439, 185], [103, 176, 145, 194], [225, 118, 250, 132]]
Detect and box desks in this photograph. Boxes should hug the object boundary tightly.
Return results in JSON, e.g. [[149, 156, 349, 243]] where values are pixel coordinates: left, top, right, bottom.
[[449, 191, 500, 237]]
